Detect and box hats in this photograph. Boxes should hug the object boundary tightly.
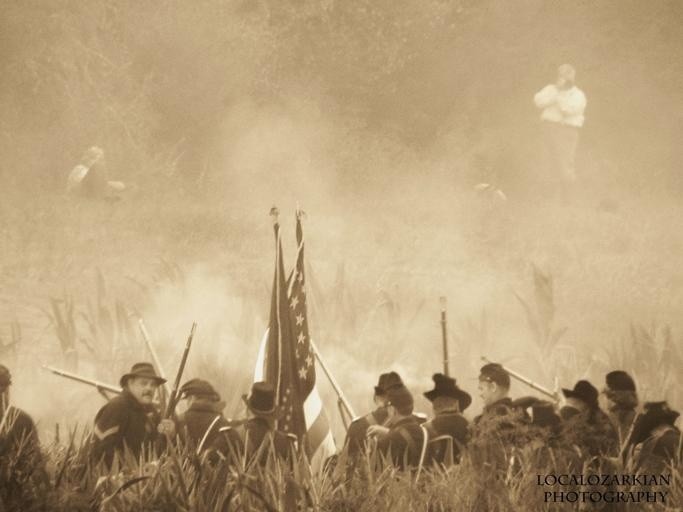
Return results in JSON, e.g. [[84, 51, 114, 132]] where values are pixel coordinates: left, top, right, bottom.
[[375, 373, 403, 394], [521, 401, 558, 424], [243, 382, 283, 419], [120, 363, 166, 389], [423, 374, 471, 412], [606, 390, 636, 409], [562, 381, 598, 405], [641, 409, 679, 427], [181, 379, 217, 396]]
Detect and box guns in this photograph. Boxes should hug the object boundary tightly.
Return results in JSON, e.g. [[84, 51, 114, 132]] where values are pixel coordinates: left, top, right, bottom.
[[136, 318, 199, 460], [481, 355, 561, 402], [41, 364, 123, 395], [308, 337, 354, 421], [440, 310, 449, 374]]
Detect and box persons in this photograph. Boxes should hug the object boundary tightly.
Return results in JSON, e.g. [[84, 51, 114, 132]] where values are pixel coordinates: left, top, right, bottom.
[[0, 364, 52, 512], [175, 377, 244, 462], [90, 362, 168, 473], [208, 381, 299, 477]]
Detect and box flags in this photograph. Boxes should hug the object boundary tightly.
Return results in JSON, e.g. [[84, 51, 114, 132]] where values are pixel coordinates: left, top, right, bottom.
[[245, 208, 338, 477]]
